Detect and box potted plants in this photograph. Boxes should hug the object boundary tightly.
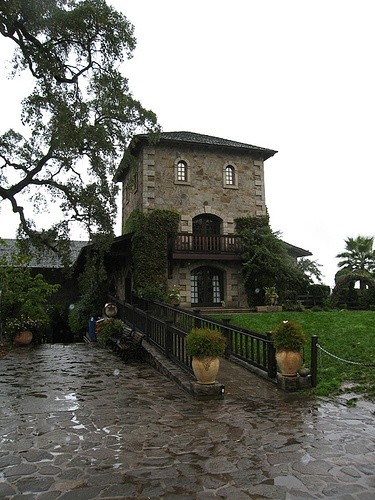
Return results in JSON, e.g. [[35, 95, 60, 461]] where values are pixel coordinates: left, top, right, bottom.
[[185, 326, 227, 384], [271, 321, 308, 377]]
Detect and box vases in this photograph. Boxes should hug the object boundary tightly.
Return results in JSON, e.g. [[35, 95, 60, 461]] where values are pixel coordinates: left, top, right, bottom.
[[14, 330, 33, 345], [169, 298, 181, 307]]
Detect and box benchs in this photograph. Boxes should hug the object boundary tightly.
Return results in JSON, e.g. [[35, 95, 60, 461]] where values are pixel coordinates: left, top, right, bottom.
[[105, 326, 145, 361]]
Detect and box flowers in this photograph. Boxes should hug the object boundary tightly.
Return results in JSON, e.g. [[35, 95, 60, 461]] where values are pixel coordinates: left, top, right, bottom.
[[168, 284, 182, 300], [1, 314, 46, 335]]
